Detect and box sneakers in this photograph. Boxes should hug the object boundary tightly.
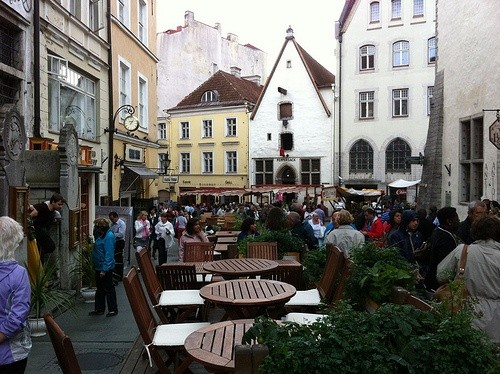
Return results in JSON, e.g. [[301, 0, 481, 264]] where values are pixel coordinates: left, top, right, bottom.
[[88, 311, 104, 316], [106, 312, 118, 317]]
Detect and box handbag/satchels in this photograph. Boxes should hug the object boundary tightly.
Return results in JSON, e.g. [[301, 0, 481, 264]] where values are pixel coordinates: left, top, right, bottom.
[[434, 243, 469, 316]]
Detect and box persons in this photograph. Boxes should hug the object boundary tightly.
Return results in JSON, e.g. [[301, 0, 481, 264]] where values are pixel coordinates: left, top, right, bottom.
[[436, 214, 500, 354], [157, 200, 328, 246], [460, 199, 500, 247], [108, 211, 126, 281], [324, 190, 438, 247], [326, 210, 365, 262], [155, 213, 175, 274], [178, 217, 214, 263], [30, 194, 66, 288], [134, 210, 151, 272], [265, 207, 287, 232], [237, 217, 260, 242], [0, 216, 33, 374], [387, 210, 431, 277], [287, 202, 316, 252], [423, 207, 465, 292], [88, 217, 118, 317], [147, 206, 159, 260]]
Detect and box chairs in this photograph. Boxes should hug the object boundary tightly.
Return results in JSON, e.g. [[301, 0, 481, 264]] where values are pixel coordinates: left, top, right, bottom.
[[184, 242, 213, 262], [285, 272, 351, 345], [247, 242, 278, 260], [155, 265, 202, 323], [200, 211, 238, 230], [283, 242, 348, 312], [135, 245, 209, 324], [43, 312, 83, 374], [123, 265, 212, 374]]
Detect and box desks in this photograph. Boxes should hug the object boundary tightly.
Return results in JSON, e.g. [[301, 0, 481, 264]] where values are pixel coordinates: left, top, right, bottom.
[[155, 259, 303, 290], [203, 258, 280, 274], [207, 231, 242, 251], [184, 318, 322, 369], [199, 278, 297, 321]]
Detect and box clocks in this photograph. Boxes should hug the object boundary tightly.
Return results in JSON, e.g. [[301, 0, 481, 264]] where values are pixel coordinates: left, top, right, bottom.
[[123, 116, 139, 132]]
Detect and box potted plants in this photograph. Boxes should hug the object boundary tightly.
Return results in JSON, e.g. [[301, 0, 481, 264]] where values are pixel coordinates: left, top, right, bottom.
[[19, 248, 82, 337], [68, 242, 124, 304]]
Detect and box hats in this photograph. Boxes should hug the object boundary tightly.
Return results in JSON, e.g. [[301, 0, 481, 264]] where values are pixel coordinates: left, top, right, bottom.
[[401, 210, 420, 234]]
